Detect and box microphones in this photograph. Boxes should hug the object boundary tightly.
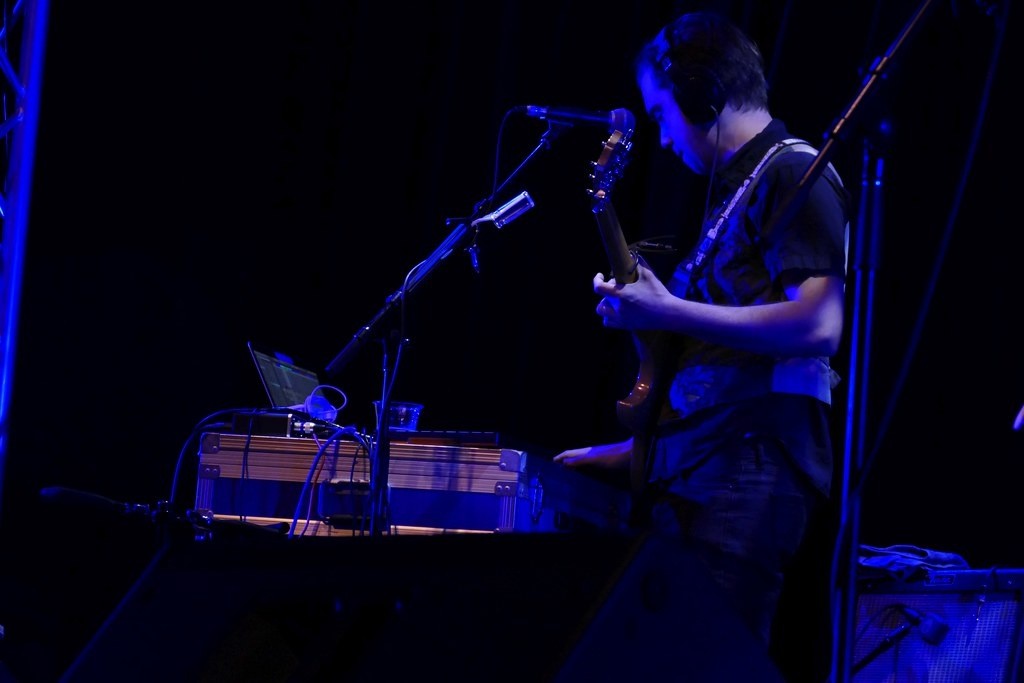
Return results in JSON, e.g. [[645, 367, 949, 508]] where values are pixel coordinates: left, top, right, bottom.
[[530, 106, 634, 136]]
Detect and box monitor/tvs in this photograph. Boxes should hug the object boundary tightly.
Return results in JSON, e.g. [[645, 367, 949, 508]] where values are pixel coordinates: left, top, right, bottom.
[[248, 340, 328, 414]]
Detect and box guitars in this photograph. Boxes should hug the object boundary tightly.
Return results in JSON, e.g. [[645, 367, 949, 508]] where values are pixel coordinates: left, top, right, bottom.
[[586, 126, 671, 471]]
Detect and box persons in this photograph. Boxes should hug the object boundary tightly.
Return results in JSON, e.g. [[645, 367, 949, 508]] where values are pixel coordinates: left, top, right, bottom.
[[550, 7, 848, 683]]
